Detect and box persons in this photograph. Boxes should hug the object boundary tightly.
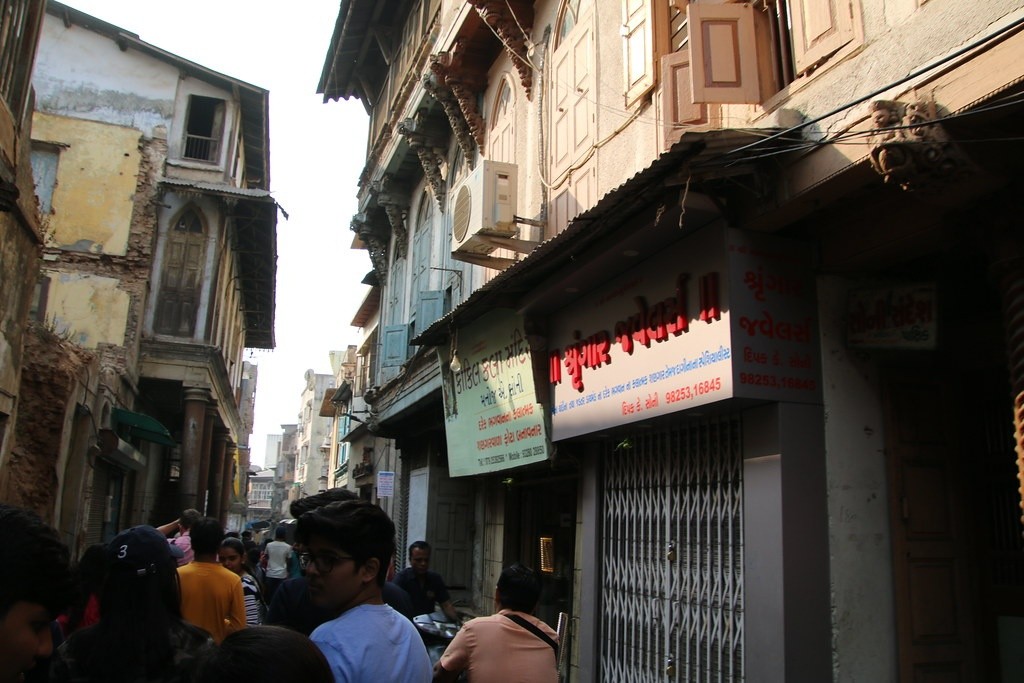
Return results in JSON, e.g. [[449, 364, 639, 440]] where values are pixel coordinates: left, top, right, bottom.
[[0, 489, 462, 683], [432, 561, 560, 682]]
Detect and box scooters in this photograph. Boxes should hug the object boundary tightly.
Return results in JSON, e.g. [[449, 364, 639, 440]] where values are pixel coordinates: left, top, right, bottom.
[[411, 613, 468, 683]]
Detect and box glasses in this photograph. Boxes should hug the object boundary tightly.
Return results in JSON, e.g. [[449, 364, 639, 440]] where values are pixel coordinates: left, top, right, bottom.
[[293, 549, 363, 575]]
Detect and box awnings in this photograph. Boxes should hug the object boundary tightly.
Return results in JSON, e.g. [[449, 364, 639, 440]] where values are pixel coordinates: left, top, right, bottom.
[[111, 408, 177, 449]]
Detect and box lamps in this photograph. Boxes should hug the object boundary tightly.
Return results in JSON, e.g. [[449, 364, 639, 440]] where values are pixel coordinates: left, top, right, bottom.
[[329, 380, 352, 405]]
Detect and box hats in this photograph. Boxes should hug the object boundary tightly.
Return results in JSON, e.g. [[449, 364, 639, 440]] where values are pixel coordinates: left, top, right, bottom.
[[102, 525, 185, 576]]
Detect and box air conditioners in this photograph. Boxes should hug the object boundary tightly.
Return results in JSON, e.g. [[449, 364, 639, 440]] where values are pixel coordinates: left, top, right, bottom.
[[448, 158, 518, 255]]
